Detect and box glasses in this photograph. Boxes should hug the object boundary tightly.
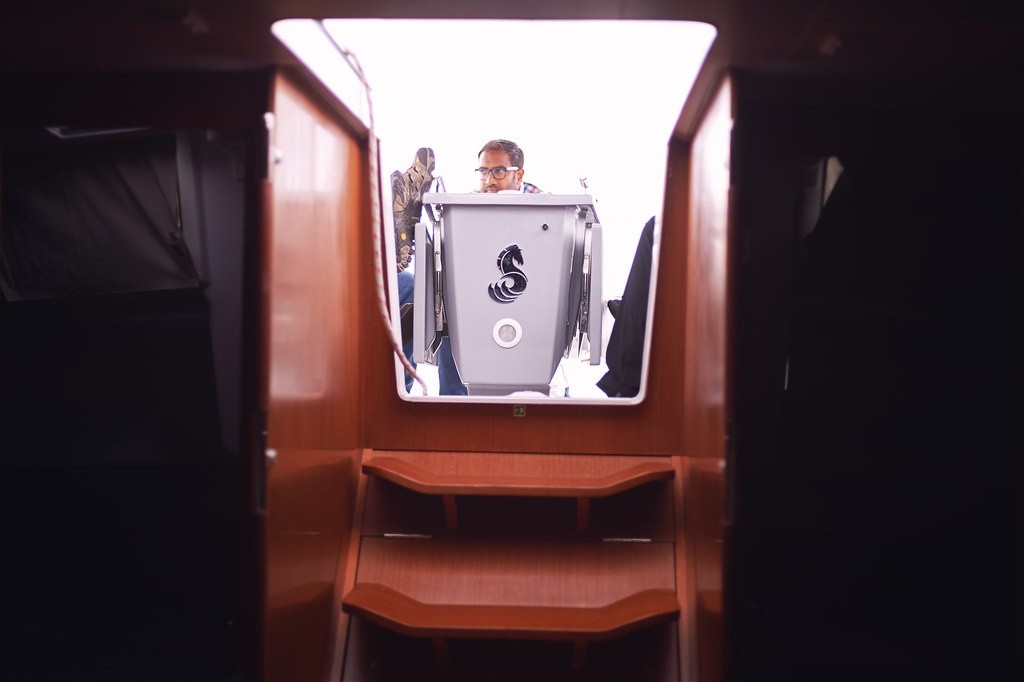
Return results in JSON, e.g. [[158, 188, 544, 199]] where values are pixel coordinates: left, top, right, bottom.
[[474, 166, 519, 180]]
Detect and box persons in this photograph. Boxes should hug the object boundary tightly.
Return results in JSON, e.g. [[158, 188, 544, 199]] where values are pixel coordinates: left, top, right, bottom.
[[437, 139, 553, 396]]
